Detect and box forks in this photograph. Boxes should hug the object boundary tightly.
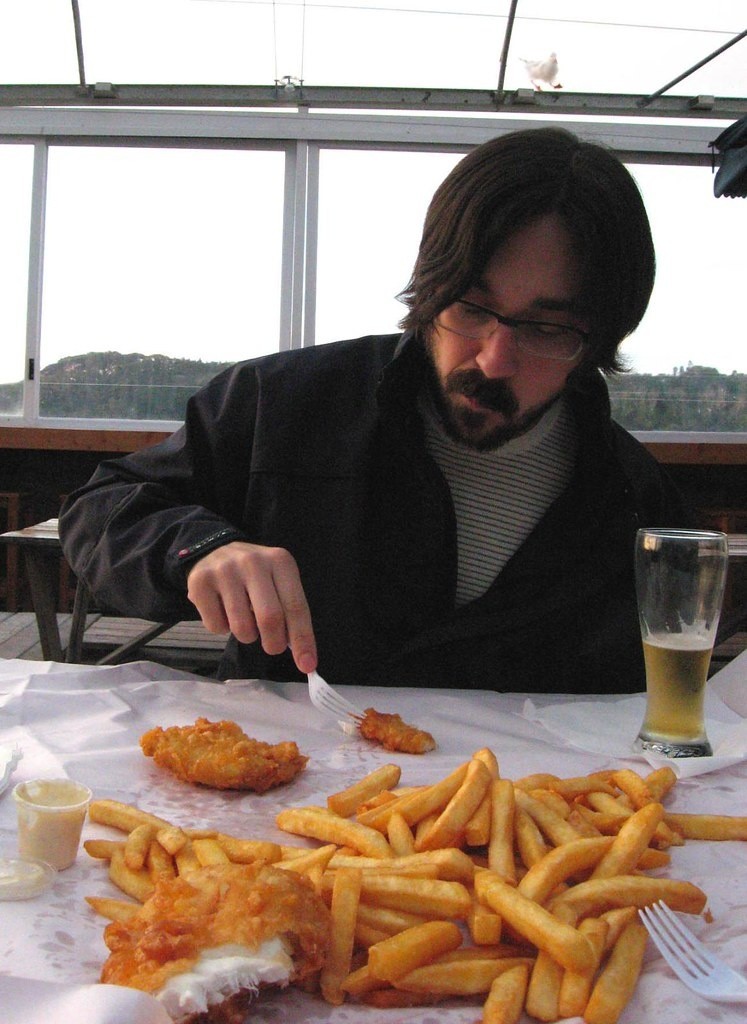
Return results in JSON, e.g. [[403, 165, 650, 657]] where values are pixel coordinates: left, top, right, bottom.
[[285, 644, 367, 728], [638, 898, 747, 1003]]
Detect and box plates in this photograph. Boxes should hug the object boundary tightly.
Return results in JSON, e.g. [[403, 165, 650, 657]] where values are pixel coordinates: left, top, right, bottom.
[[0, 855, 58, 902]]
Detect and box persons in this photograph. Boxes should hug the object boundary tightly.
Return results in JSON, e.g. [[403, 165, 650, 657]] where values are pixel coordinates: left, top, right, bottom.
[[57, 125, 700, 696]]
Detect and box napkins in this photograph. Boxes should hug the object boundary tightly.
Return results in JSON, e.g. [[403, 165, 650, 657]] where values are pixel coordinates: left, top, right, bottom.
[[514, 683, 746, 779]]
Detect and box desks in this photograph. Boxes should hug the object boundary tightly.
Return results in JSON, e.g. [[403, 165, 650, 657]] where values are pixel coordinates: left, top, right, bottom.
[[0, 518, 747, 666]]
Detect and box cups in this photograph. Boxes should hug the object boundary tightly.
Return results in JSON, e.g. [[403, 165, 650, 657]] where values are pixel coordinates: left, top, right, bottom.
[[14, 777, 92, 871], [634, 529, 728, 759]]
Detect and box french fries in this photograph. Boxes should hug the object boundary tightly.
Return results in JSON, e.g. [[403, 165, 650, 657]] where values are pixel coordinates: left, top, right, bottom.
[[82, 747, 747, 1024]]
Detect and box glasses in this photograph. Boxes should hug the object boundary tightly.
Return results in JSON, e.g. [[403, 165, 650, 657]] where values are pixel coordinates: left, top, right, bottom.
[[435, 299, 592, 361]]
